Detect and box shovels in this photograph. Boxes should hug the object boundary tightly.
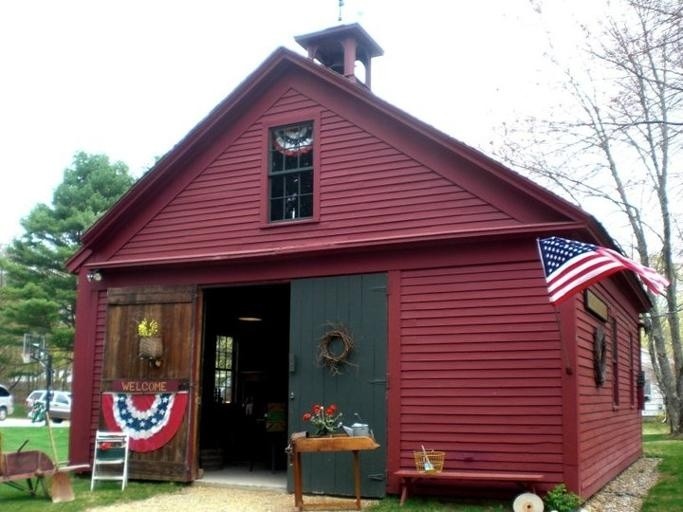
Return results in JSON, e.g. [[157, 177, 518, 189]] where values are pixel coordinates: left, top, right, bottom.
[[45, 411, 75, 504]]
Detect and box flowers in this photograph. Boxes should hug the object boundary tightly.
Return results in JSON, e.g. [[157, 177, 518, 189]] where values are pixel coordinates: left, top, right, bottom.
[[302, 403, 344, 433]]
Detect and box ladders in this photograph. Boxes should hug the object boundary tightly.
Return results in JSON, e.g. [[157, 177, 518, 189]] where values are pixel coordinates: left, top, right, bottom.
[[91, 430, 129, 492]]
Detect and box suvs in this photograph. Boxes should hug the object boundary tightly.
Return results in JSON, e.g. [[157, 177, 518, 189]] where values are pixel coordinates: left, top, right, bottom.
[[0, 385, 15, 422]]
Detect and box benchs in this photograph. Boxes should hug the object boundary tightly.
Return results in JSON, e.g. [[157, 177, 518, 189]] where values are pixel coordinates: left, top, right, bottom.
[[392, 470, 544, 507]]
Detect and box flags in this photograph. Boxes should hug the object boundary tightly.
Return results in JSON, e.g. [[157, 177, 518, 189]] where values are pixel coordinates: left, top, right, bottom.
[[535, 235, 674, 310]]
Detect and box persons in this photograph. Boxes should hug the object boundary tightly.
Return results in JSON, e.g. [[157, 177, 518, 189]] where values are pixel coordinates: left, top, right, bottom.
[[28, 398, 45, 422]]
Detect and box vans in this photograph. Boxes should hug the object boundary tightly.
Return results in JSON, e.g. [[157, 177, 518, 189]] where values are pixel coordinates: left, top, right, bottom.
[[26, 388, 72, 424]]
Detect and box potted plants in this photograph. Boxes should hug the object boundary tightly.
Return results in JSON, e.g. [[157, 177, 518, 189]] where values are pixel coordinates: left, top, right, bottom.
[[136, 317, 164, 360]]
